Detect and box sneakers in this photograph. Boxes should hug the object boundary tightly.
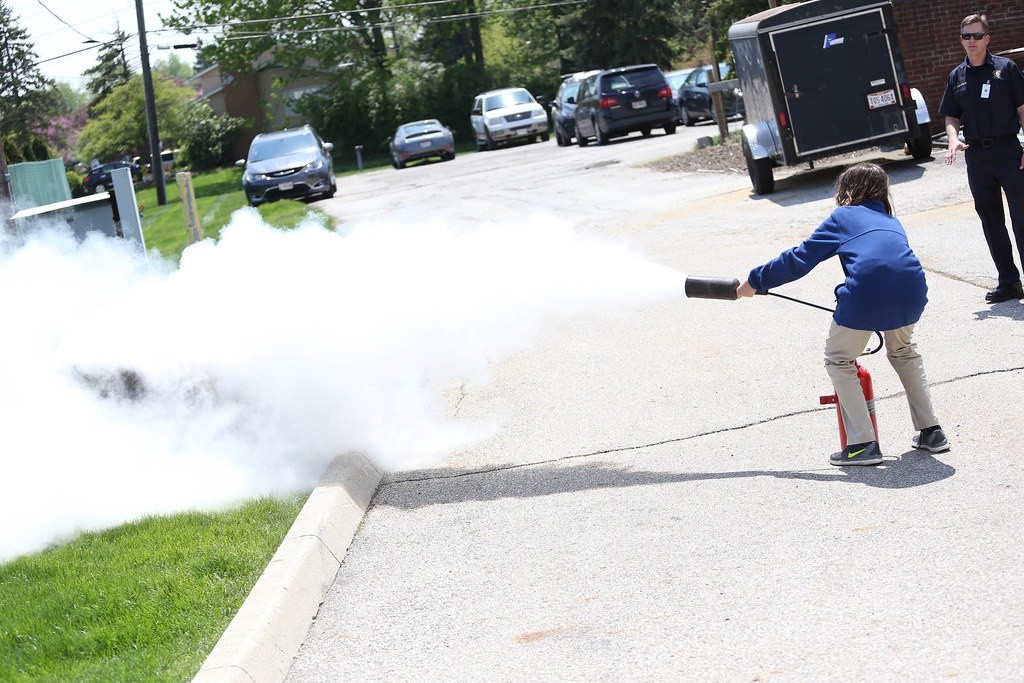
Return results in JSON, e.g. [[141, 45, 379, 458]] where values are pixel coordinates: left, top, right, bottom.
[[911, 429, 950, 452], [830, 442, 883, 465]]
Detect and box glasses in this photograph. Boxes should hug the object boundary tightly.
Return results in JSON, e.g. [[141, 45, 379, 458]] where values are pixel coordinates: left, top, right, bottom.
[[960, 33, 986, 40]]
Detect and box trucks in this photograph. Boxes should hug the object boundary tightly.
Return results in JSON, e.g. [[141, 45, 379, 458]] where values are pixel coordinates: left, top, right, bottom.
[[727, 1, 934, 194]]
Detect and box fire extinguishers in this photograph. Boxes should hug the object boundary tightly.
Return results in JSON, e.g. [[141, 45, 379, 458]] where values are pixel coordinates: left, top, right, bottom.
[[683, 275, 884, 452]]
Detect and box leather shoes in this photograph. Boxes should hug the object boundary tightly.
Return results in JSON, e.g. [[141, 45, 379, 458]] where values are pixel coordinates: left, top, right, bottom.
[[985, 281, 1024, 301]]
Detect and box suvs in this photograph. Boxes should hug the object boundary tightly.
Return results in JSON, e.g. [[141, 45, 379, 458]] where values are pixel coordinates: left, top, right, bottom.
[[81, 161, 151, 195], [233, 123, 338, 209], [548, 69, 604, 145]]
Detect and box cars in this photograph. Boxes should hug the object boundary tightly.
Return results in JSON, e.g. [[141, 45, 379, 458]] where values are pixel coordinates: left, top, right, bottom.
[[676, 63, 746, 126], [573, 64, 677, 146], [469, 86, 550, 150], [131, 154, 153, 168], [387, 118, 456, 169], [664, 66, 696, 100], [161, 149, 181, 171]]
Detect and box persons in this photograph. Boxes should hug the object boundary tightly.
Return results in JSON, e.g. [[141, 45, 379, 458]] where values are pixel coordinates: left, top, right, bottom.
[[734, 161, 951, 467], [936, 12, 1024, 302]]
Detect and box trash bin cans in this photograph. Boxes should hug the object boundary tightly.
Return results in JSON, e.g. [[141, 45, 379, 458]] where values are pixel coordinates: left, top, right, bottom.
[[11, 191, 115, 249]]
